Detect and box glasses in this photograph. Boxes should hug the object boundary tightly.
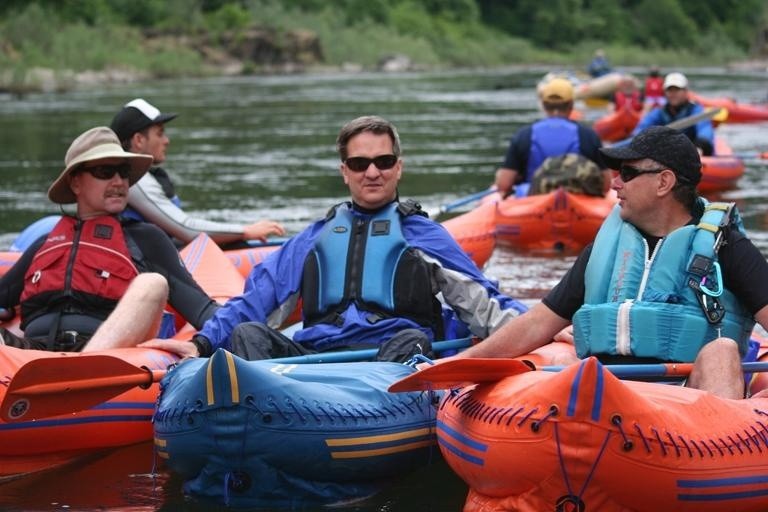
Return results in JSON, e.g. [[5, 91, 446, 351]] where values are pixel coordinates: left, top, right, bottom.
[[76, 163, 132, 179], [618, 165, 664, 183], [344, 155, 398, 172]]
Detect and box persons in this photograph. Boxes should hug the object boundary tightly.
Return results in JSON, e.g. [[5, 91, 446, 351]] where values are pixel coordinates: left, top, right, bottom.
[[494, 74, 612, 196], [415, 126, 767, 398], [591, 47, 611, 76], [642, 66, 668, 108], [136, 116, 574, 362], [113, 99, 285, 249], [0, 128, 221, 351], [631, 73, 717, 157], [615, 79, 642, 111]]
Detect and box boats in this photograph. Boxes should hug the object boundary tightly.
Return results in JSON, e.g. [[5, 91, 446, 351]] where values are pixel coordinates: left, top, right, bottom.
[[0, 232, 246, 482], [0, 197, 496, 326], [153, 315, 464, 507], [436, 316, 768, 507]]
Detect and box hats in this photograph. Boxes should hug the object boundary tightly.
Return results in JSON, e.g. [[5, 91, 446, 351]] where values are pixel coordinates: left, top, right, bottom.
[[543, 78, 575, 103], [47, 126, 154, 204], [663, 72, 688, 91], [598, 127, 703, 185], [111, 99, 180, 141]]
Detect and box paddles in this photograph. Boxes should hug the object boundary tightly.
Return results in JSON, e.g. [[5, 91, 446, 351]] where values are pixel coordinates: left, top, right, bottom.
[[389, 357, 767, 393], [443, 108, 720, 211], [0, 337, 486, 423]]
